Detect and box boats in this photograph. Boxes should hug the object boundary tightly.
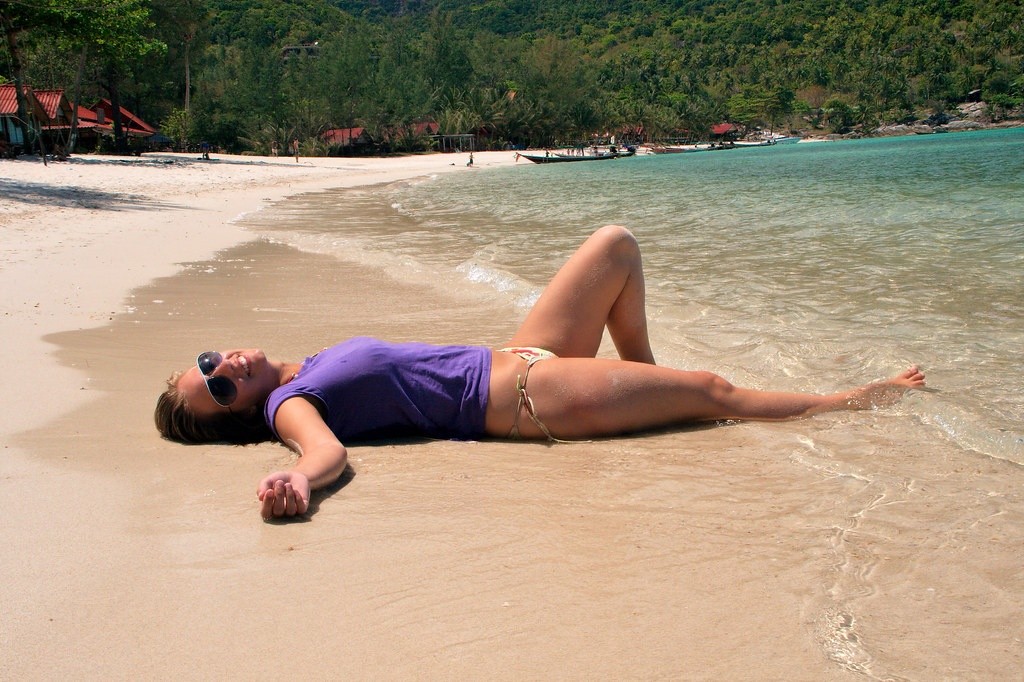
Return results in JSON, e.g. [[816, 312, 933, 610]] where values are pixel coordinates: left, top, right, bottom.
[[724, 137, 803, 149], [517, 147, 637, 163], [650, 145, 724, 154]]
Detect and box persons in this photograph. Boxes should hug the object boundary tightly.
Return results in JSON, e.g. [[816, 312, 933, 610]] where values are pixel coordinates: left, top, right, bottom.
[[200, 140, 210, 160], [153, 227, 926, 518], [294, 137, 299, 162], [545, 150, 549, 157], [469, 152, 474, 164]]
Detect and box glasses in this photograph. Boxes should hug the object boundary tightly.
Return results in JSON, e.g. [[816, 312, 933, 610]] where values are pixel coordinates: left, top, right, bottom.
[[197, 350, 237, 415]]
